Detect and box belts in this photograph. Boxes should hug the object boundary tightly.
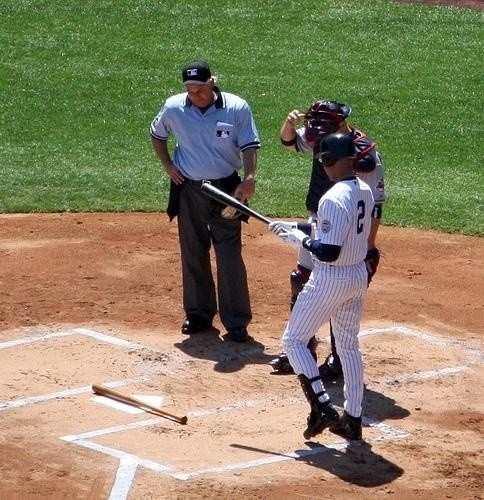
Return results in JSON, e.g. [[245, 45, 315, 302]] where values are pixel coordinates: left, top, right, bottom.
[[178, 170, 240, 189]]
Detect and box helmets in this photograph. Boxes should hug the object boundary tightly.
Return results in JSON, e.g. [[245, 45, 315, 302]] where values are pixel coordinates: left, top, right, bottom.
[[314, 132, 358, 159], [302, 98, 351, 144]]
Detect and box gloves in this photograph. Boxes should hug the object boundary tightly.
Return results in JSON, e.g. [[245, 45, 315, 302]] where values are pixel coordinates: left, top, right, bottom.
[[268, 220, 310, 249]]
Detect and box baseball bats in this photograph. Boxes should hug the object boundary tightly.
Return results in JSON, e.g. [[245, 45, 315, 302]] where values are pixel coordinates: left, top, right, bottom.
[[200, 183, 288, 233], [92, 383, 187, 424]]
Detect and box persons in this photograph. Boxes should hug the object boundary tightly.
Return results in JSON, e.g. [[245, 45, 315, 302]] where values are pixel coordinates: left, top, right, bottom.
[[268, 131, 369, 441], [147, 61, 261, 339], [269, 100, 386, 379]]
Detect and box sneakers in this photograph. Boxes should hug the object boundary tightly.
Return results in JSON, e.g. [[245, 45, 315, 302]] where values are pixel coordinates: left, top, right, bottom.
[[268, 346, 318, 374], [301, 400, 340, 440], [329, 409, 363, 441], [318, 351, 343, 381]]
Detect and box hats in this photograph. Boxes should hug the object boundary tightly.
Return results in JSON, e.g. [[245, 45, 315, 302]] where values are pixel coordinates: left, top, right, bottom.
[[181, 59, 212, 86]]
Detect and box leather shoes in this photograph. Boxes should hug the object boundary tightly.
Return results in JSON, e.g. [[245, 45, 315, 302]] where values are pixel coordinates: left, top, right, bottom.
[[229, 325, 249, 343], [180, 314, 213, 333]]
